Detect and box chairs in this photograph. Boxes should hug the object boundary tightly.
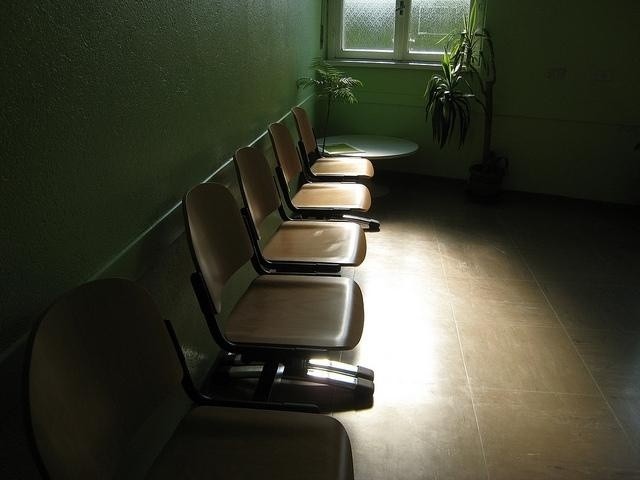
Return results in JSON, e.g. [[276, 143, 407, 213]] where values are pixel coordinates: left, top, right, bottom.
[[233, 147, 366, 266], [269, 122, 380, 228], [186, 183, 374, 394], [292, 106, 374, 178], [28, 277, 353, 480]]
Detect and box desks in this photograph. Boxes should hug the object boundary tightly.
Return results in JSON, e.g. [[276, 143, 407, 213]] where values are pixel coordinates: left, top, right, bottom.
[[317, 134, 419, 161]]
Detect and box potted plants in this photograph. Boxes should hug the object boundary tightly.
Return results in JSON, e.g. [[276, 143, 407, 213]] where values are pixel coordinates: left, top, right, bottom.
[[424, 0, 512, 231]]
